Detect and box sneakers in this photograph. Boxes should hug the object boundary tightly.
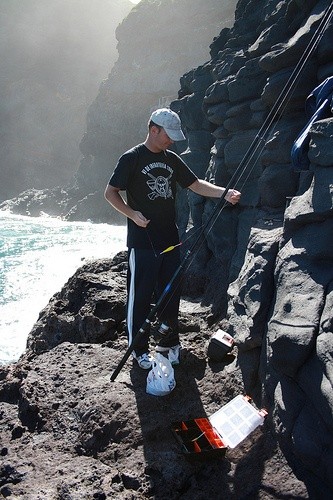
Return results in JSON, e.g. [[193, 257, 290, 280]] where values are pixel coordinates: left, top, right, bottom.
[[131, 352, 151, 370], [168, 344, 181, 366]]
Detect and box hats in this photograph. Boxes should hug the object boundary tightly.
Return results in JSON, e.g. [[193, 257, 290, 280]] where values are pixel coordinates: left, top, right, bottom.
[[151, 106, 186, 142]]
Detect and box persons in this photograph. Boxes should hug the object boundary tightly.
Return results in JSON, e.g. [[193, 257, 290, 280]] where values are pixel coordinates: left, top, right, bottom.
[[104, 107, 241, 369]]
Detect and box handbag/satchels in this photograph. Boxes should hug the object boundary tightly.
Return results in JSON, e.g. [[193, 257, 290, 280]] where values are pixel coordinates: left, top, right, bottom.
[[146, 351, 176, 397]]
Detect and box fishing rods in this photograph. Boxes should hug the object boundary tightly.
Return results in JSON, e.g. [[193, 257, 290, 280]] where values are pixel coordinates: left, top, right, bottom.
[[110, 1, 333, 382]]
